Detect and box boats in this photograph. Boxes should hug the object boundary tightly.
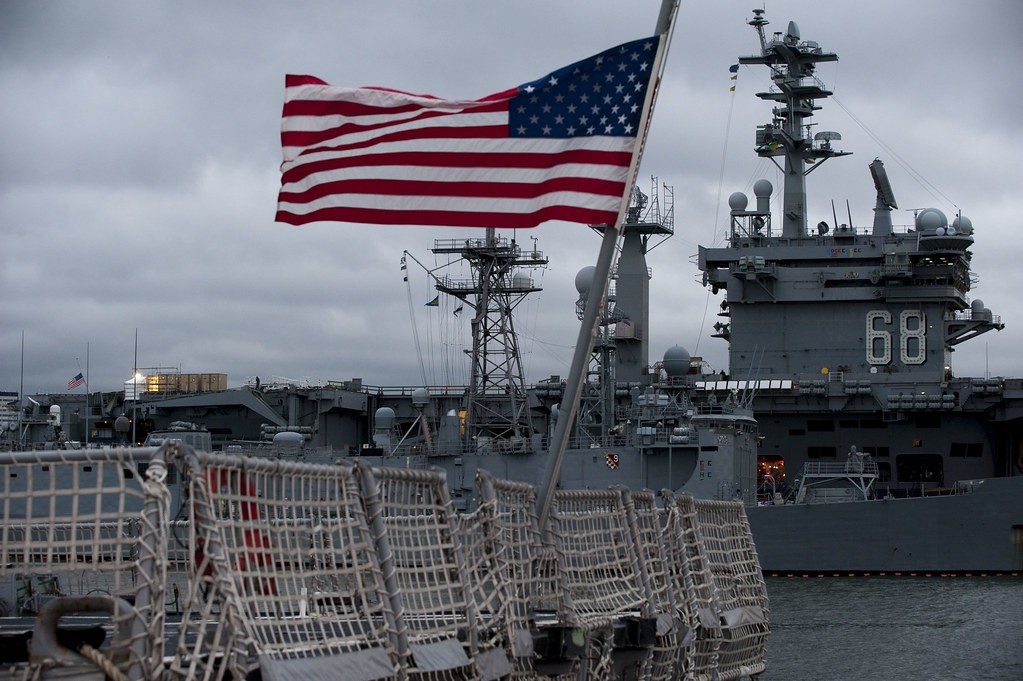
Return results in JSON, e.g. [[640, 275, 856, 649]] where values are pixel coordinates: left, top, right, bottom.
[[0, 0, 1023, 582]]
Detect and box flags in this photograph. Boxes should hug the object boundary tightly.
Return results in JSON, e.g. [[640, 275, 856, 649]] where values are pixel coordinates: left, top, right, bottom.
[[67, 372, 85, 390], [273, 31, 667, 230]]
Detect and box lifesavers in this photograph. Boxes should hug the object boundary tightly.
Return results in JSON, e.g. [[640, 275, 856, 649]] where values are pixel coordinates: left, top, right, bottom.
[[192, 462, 264, 590]]
